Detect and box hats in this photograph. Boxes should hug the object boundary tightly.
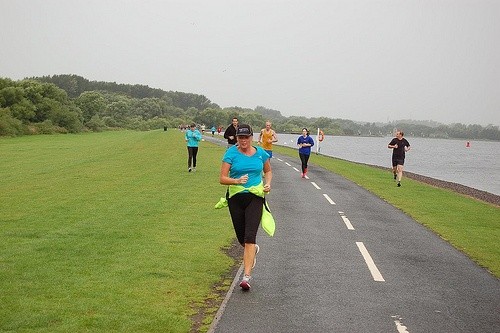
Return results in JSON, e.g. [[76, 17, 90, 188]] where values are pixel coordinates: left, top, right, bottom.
[[236, 124, 253, 136]]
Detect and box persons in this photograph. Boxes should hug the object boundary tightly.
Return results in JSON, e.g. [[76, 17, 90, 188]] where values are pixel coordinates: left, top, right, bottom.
[[296, 128, 314, 180], [211, 127, 215, 137], [388, 132, 411, 186], [214, 124, 275, 288], [258, 122, 278, 177], [178, 124, 206, 133], [185, 123, 202, 172], [223, 117, 241, 149], [218, 125, 222, 134]]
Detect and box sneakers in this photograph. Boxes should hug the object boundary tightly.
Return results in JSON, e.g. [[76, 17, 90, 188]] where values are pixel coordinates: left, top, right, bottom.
[[251, 244, 259, 269], [239, 274, 251, 289]]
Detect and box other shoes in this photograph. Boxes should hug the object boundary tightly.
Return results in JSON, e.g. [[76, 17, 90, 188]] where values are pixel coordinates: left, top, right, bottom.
[[303, 168, 307, 173], [188, 168, 192, 172], [394, 174, 397, 179], [302, 174, 305, 177], [192, 167, 196, 172], [397, 182, 401, 187]]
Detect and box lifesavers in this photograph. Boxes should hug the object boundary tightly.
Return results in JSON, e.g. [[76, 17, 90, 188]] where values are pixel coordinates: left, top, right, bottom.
[[319, 129, 325, 142]]
